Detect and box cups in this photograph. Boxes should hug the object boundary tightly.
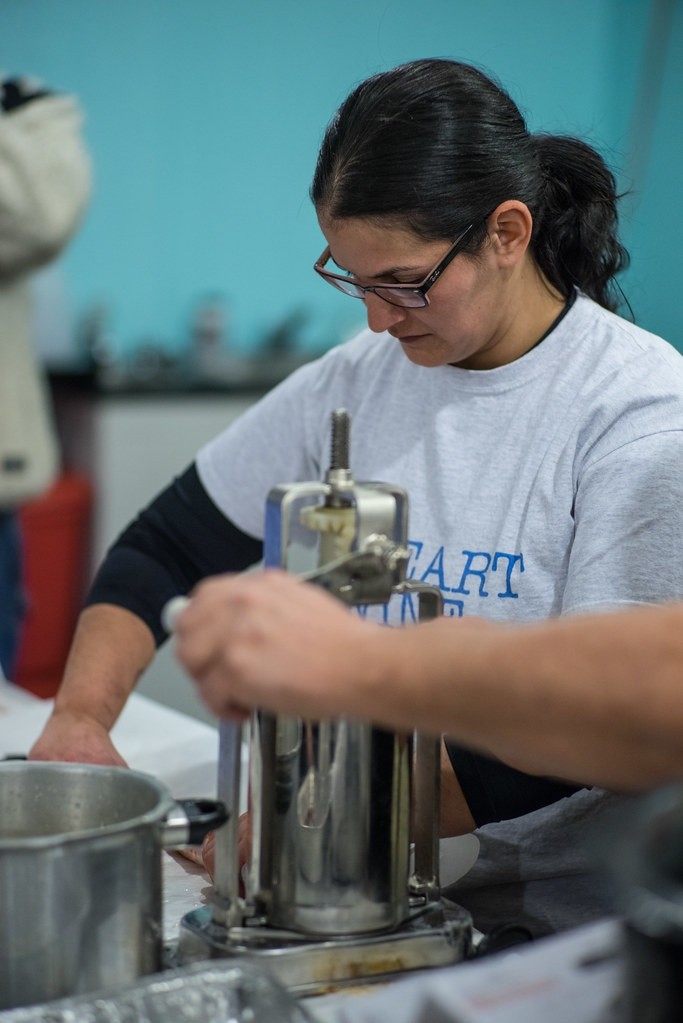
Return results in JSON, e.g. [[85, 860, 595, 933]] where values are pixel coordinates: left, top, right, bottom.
[[213, 698, 408, 934]]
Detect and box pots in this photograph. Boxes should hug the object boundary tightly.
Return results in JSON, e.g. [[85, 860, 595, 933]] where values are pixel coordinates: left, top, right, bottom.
[[0, 760, 233, 1011]]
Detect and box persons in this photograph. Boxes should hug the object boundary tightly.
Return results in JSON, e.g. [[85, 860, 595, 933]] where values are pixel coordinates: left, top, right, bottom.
[[177, 567, 679, 792], [24, 58, 682, 897], [0, 79, 61, 681]]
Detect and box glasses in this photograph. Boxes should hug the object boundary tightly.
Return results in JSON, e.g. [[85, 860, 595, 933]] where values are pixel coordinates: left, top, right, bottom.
[[311, 207, 493, 314]]
